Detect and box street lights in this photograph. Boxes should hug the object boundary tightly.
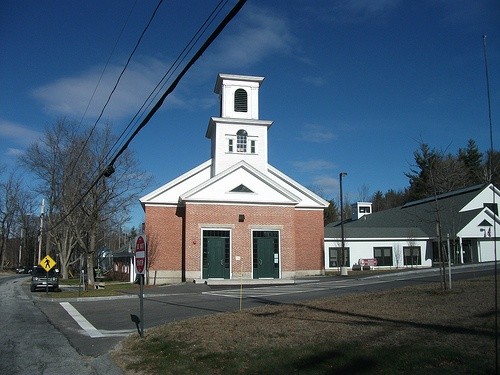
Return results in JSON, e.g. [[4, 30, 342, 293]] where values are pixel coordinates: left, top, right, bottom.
[[339, 172, 347, 277]]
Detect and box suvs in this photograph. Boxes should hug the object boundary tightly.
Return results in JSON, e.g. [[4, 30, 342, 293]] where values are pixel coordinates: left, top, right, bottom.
[[30, 265, 59, 292]]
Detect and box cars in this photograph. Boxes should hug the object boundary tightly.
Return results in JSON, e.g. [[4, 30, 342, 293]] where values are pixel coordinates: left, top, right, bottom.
[[16, 266, 28, 274]]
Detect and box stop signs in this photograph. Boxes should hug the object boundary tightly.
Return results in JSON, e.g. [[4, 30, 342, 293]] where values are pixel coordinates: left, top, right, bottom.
[[134, 235, 146, 274]]
